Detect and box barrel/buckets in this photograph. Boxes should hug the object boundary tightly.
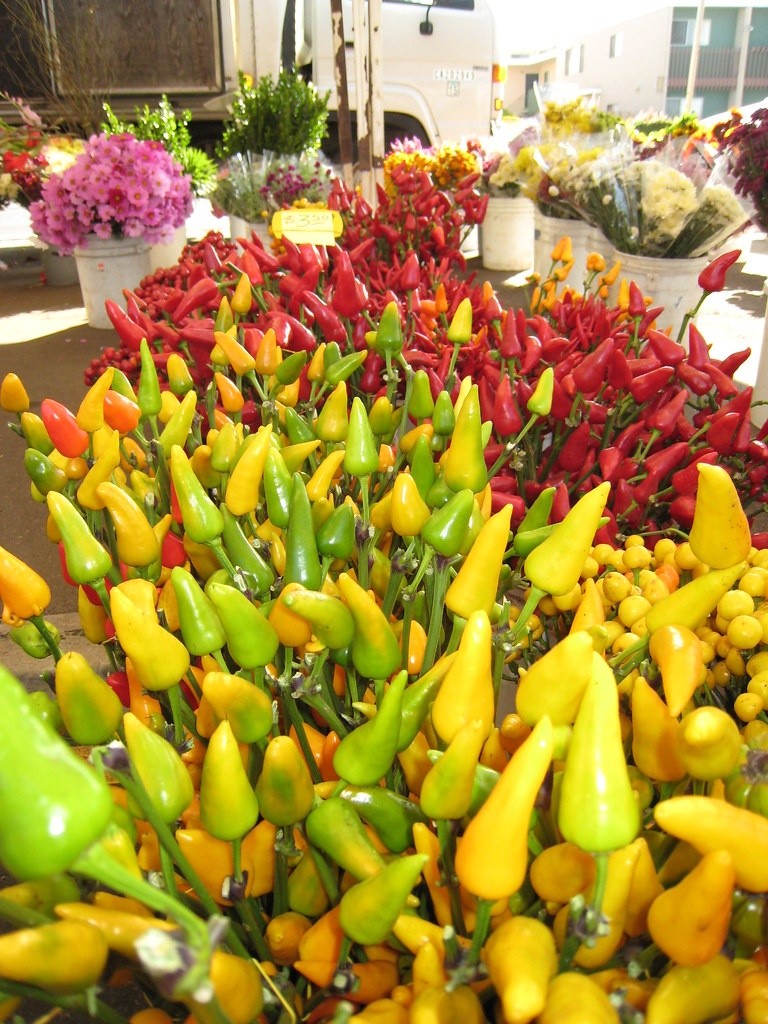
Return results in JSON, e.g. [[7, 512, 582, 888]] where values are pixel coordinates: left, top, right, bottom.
[[232, 215, 273, 253], [749, 280, 768, 430], [40, 224, 186, 339], [476, 197, 707, 354]]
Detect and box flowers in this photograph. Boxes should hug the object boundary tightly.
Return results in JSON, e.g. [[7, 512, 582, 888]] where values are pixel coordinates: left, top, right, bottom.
[[0, 0, 768, 259]]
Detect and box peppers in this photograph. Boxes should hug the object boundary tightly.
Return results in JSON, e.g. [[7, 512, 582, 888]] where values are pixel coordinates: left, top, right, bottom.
[[0, 156, 768, 1024]]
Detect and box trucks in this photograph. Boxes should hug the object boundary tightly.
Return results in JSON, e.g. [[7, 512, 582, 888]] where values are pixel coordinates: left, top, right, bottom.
[[1, 0, 502, 173]]
